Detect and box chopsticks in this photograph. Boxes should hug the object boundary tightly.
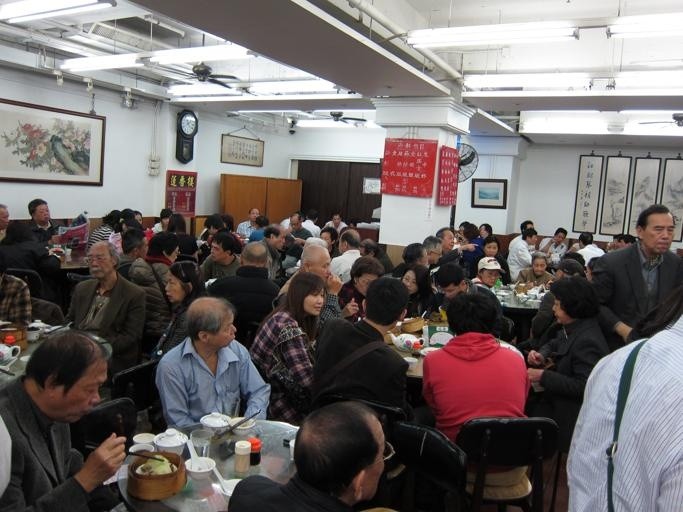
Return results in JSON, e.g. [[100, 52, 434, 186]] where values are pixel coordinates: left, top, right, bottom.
[[79, 438, 166, 463]]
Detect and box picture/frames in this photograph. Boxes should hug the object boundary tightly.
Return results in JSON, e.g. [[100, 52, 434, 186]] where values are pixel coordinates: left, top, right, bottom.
[[220, 134, 264, 167], [572, 154, 604, 234], [627, 156, 662, 238], [599, 155, 633, 236], [0, 98, 107, 186], [659, 157, 683, 243], [471, 178, 508, 209]]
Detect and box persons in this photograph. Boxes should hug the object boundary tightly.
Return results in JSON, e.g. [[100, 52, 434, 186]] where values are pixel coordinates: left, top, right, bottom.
[[509, 221, 536, 251], [167, 213, 197, 254], [423, 290, 530, 487], [203, 217, 242, 254], [258, 224, 286, 280], [324, 213, 348, 232], [0, 263, 33, 323], [120, 229, 146, 279], [576, 232, 606, 266], [567, 242, 580, 253], [87, 211, 120, 250], [436, 229, 456, 251], [527, 278, 609, 427], [515, 256, 555, 284], [129, 232, 179, 338], [403, 244, 428, 266], [281, 212, 312, 267], [461, 225, 485, 265], [248, 271, 326, 426], [607, 234, 624, 251], [207, 241, 281, 348], [0, 331, 179, 511], [29, 199, 63, 244], [249, 216, 269, 242], [360, 239, 395, 272], [592, 205, 683, 351], [432, 262, 503, 322], [221, 213, 244, 243], [315, 278, 414, 421], [586, 257, 601, 280], [454, 221, 469, 241], [531, 259, 584, 337], [0, 204, 9, 241], [301, 210, 321, 237], [400, 267, 436, 317], [229, 401, 395, 511], [65, 242, 146, 365], [350, 221, 357, 229], [155, 298, 271, 433], [339, 256, 385, 322], [151, 209, 173, 233], [236, 208, 260, 239], [333, 228, 351, 257], [565, 315, 682, 512], [539, 227, 566, 258], [304, 237, 329, 248], [330, 230, 361, 284], [471, 256, 501, 291], [149, 260, 204, 358], [478, 223, 493, 240], [108, 208, 135, 255], [560, 252, 585, 267], [483, 236, 510, 284], [271, 244, 344, 328], [0, 220, 60, 302], [320, 226, 342, 258], [134, 210, 143, 225], [422, 236, 478, 268], [200, 237, 241, 281], [506, 228, 538, 283], [619, 236, 635, 249], [280, 215, 291, 228]]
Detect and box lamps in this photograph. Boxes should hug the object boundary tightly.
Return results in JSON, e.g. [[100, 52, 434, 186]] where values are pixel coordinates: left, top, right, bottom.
[[1, 1, 118, 24], [150, 44, 258, 65], [60, 53, 145, 73], [403, 20, 582, 51]]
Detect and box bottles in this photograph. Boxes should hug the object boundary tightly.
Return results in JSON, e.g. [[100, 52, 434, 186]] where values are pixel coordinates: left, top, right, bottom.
[[248, 437, 262, 465], [235, 441, 251, 472], [495, 279, 501, 291]]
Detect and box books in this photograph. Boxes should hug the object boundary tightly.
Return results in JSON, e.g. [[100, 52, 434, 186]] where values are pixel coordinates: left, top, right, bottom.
[[58, 210, 90, 248]]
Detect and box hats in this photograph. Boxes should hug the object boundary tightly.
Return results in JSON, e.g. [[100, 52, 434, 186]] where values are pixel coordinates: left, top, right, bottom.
[[549, 259, 586, 277], [478, 256, 502, 272]]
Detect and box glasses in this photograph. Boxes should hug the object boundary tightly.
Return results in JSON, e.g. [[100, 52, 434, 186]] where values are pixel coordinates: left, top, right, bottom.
[[175, 248, 181, 258], [364, 439, 397, 468], [429, 250, 444, 259], [403, 274, 418, 286], [289, 223, 300, 228], [358, 277, 378, 285], [84, 256, 107, 264]]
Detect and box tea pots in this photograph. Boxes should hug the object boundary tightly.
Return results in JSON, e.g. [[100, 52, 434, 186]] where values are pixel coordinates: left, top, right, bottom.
[[389, 330, 428, 354], [0, 343, 22, 366]]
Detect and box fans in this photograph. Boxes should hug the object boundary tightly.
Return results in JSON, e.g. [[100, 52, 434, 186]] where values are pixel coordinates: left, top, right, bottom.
[[155, 62, 238, 90], [312, 112, 367, 124], [455, 144, 478, 183]]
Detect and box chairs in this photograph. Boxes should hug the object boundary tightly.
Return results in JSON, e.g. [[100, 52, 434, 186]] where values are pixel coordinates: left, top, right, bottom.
[[86, 398, 138, 437], [385, 423, 468, 512], [456, 417, 563, 512], [112, 361, 159, 411], [330, 394, 405, 434], [5, 268, 45, 299]]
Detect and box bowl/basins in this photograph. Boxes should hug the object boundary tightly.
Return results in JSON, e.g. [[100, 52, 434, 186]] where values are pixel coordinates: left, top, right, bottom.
[[406, 355, 421, 372], [183, 457, 215, 482], [131, 431, 158, 443], [25, 325, 41, 341], [232, 415, 258, 437], [210, 478, 250, 501], [125, 442, 157, 459]]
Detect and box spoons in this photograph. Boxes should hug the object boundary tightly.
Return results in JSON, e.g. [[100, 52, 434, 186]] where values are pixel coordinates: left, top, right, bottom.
[[212, 467, 232, 494], [185, 438, 207, 472]]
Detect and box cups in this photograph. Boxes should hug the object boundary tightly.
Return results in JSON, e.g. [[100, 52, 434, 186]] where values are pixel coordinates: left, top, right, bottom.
[[155, 427, 187, 461], [195, 413, 234, 439]]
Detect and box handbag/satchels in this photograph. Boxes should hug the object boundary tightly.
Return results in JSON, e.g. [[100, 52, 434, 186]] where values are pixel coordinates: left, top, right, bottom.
[[308, 339, 388, 394], [149, 258, 175, 314], [271, 322, 317, 412], [607, 337, 648, 511]]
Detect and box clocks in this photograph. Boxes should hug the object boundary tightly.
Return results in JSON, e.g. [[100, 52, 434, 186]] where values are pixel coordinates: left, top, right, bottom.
[[175, 109, 199, 164]]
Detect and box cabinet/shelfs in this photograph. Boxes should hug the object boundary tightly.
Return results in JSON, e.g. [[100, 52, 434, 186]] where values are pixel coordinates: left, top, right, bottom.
[[339, 227, 379, 243], [220, 173, 302, 227]]
